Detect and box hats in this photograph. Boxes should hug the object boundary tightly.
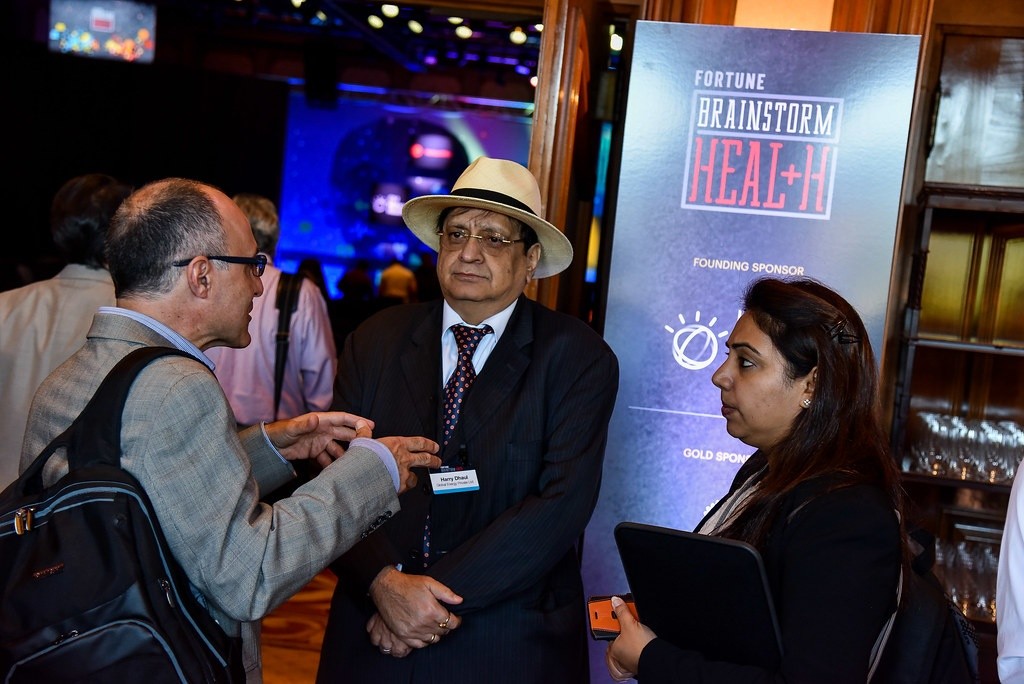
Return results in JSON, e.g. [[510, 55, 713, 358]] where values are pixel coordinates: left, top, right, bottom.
[[401, 156, 574, 280]]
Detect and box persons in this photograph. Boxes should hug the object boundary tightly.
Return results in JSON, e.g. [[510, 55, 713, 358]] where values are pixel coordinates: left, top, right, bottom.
[[995, 456, 1024, 684], [605, 275, 981, 684], [0, 172, 443, 684], [311, 156, 622, 684]]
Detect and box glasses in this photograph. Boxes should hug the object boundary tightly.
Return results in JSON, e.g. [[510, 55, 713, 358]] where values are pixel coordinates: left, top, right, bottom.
[[434, 226, 523, 257], [172, 254, 268, 278]]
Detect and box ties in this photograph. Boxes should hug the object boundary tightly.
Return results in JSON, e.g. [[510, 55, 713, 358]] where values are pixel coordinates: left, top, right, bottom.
[[422, 324, 495, 570]]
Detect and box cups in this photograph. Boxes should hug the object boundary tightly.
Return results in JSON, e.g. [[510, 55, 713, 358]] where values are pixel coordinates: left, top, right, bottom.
[[912, 411, 1024, 482], [933, 536, 998, 625]]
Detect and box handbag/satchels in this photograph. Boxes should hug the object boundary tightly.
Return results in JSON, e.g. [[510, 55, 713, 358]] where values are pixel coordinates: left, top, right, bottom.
[[861, 510, 979, 684], [259, 272, 350, 506], [0, 345, 248, 684]]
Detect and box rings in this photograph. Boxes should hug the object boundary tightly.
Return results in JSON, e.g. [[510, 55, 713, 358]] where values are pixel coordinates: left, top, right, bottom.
[[428, 633, 436, 644], [438, 611, 452, 630], [382, 648, 392, 652]]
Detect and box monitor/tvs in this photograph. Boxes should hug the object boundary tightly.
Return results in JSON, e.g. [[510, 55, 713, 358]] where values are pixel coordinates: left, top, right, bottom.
[[47, 0, 157, 64]]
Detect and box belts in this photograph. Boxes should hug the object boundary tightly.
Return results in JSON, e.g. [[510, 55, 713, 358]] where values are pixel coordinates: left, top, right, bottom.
[[406, 549, 450, 565]]
